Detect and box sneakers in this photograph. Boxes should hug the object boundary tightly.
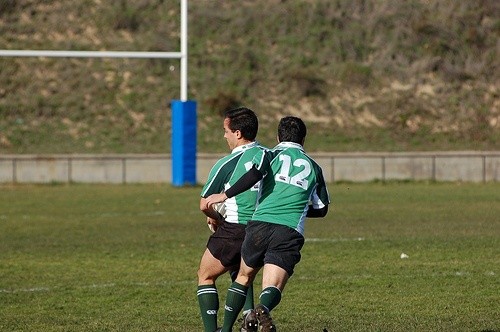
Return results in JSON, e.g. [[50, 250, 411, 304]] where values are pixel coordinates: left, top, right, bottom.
[[255, 305, 276, 332], [238, 310, 258, 332]]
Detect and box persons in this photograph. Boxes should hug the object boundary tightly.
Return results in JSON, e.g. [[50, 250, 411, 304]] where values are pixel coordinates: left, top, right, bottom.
[[205, 116, 330, 332], [197, 107, 272, 332]]
